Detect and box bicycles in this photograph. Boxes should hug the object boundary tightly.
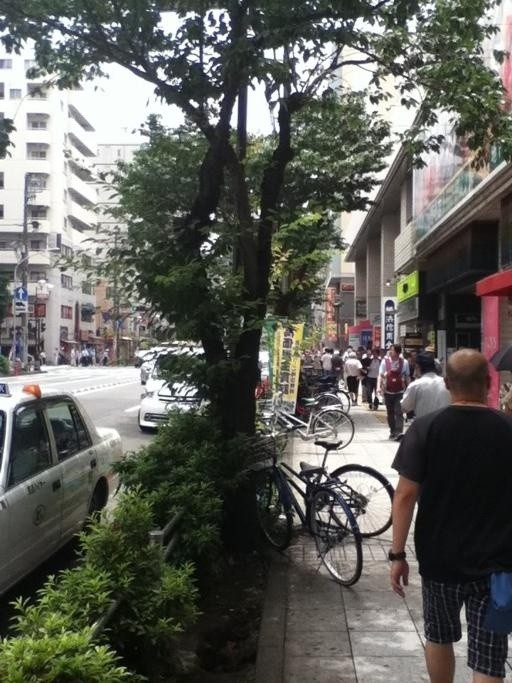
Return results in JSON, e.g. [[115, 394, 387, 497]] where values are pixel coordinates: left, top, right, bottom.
[[255, 371, 395, 586]]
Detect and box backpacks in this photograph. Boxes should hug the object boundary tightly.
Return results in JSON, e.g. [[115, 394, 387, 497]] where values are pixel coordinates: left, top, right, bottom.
[[385, 358, 405, 391]]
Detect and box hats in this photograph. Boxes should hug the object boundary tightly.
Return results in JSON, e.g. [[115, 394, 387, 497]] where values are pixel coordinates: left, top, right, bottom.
[[416, 352, 434, 366], [483, 572, 512, 636]]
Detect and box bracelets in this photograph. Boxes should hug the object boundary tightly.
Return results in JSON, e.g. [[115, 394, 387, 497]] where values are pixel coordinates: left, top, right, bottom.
[[389, 550, 406, 561]]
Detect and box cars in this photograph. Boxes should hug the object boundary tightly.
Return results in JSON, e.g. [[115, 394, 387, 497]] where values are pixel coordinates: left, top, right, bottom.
[[134, 341, 271, 436], [0, 383, 123, 595]]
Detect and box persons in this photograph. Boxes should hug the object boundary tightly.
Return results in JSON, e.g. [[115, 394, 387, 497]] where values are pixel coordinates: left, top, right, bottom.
[[388, 348, 511, 683], [39, 352, 46, 364], [103, 348, 109, 366], [401, 352, 450, 417], [70, 346, 96, 367], [321, 346, 442, 439]]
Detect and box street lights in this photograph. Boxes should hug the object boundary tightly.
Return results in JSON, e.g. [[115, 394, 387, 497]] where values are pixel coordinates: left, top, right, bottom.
[[0, 220, 42, 229], [33, 279, 53, 371], [12, 247, 64, 368]]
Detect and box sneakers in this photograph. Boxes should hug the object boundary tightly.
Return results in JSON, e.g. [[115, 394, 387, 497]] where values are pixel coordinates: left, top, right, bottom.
[[389, 432, 403, 441], [351, 399, 378, 410]]
